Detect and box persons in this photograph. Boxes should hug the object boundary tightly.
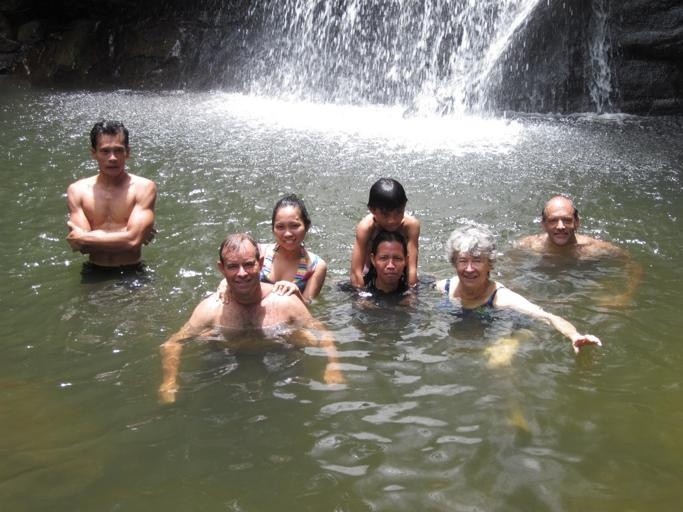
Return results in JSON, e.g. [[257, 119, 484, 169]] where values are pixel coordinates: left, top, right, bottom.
[[336, 176, 439, 295], [64, 119, 159, 284], [216, 192, 328, 309], [512, 195, 644, 312], [355, 230, 410, 309], [154, 231, 347, 401], [428, 225, 604, 357]]
